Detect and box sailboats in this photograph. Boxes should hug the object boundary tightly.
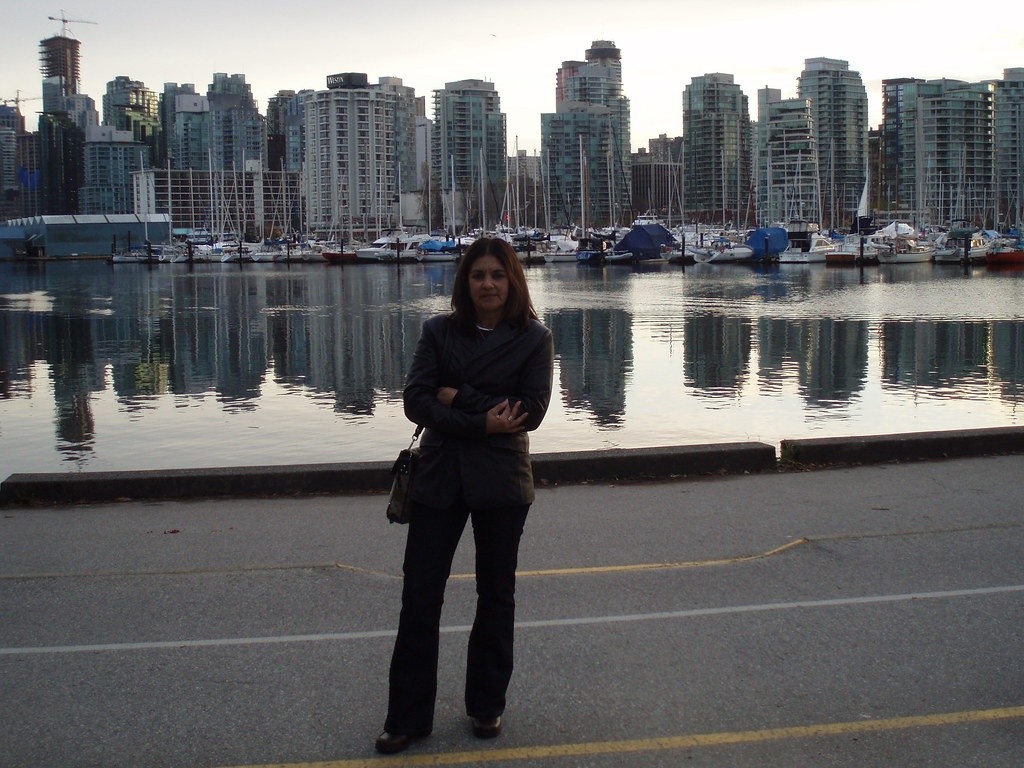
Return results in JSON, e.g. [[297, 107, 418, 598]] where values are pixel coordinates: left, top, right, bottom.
[[110, 133, 1024, 266]]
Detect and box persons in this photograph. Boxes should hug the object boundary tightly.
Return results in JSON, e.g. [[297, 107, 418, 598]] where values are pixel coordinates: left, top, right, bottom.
[[375, 238, 553, 754]]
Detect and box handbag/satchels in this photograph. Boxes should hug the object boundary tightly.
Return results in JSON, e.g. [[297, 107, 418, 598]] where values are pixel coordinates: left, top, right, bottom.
[[385, 448, 420, 524]]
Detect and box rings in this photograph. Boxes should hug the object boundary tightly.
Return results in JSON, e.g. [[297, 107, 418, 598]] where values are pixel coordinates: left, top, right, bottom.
[[508, 416, 513, 422]]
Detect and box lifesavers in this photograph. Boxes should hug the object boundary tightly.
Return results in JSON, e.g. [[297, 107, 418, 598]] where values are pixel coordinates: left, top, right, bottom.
[[720, 247, 724, 253]]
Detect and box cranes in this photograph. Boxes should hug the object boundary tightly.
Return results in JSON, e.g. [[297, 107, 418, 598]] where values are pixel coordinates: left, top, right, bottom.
[[48, 9, 98, 38], [2, 90, 43, 134]]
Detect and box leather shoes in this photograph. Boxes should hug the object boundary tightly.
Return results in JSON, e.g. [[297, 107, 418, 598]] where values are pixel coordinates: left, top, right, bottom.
[[472, 716, 501, 739], [375, 731, 416, 754]]
